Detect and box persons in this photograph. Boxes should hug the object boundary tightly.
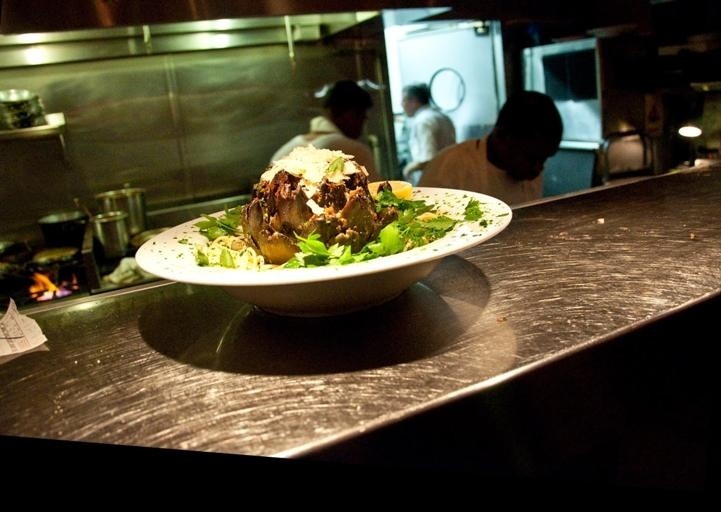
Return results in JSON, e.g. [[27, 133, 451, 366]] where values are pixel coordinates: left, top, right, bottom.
[[397, 80, 457, 188], [605, 81, 706, 178], [268, 75, 383, 183], [412, 90, 564, 207]]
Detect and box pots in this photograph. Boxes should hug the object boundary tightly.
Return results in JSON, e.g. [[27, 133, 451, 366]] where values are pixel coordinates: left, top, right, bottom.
[[38, 187, 148, 268]]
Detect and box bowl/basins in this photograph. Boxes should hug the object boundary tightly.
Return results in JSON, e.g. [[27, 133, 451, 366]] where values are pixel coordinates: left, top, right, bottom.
[[0, 89, 46, 129], [135, 185, 514, 313]]
